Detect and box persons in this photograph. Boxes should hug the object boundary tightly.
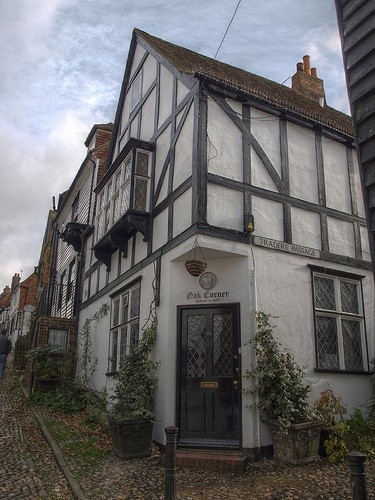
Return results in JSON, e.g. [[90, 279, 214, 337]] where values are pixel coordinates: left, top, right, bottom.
[[0, 329, 11, 380]]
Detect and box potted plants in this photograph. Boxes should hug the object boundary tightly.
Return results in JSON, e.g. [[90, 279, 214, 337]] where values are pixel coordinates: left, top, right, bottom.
[[110, 330, 162, 459], [26, 341, 72, 392], [242, 309, 322, 467], [311, 383, 350, 464], [85, 385, 116, 431]]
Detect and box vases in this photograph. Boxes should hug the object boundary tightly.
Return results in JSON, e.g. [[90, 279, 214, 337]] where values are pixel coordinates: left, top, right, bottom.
[[184, 238, 208, 278]]
